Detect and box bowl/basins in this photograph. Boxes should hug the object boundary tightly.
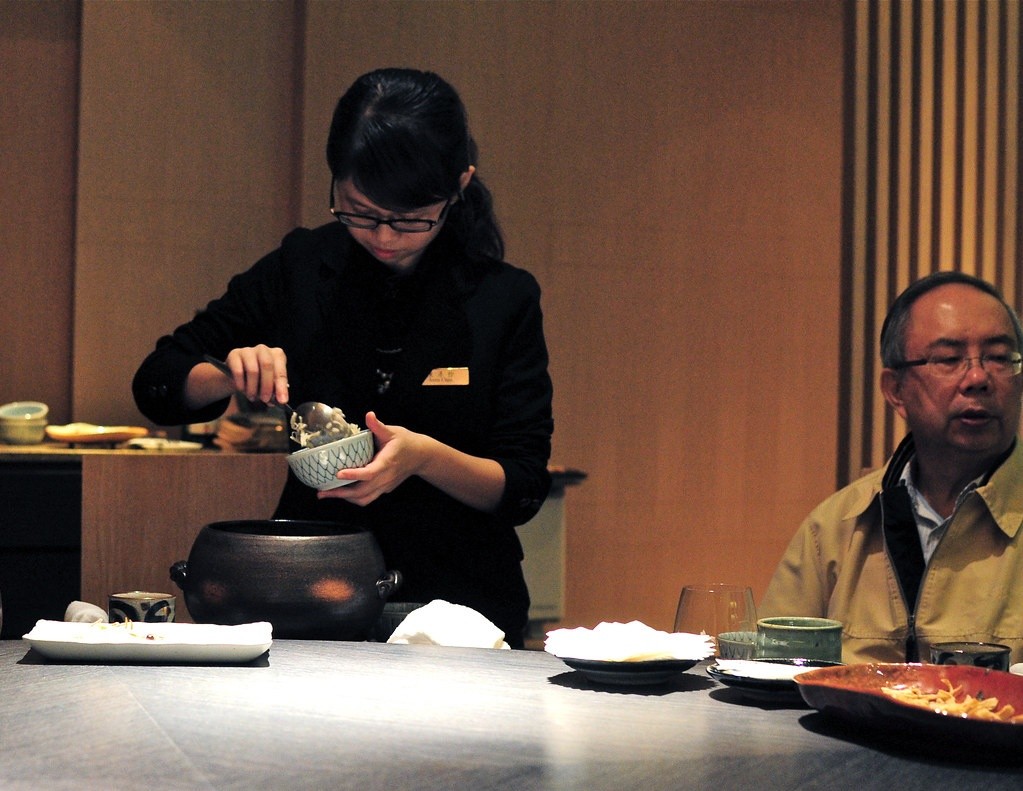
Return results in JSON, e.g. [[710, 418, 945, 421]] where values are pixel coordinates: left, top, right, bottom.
[[286, 428, 374, 491], [716, 631, 758, 658], [0, 400, 49, 423], [0, 421, 48, 444]]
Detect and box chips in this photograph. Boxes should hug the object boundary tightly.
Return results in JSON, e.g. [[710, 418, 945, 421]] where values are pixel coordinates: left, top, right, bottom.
[[878, 677, 1023, 724]]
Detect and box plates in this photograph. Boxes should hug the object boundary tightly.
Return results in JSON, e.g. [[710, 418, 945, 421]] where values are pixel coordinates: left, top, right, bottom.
[[128, 438, 203, 451], [707, 658, 845, 700], [543, 634, 712, 686], [23, 618, 272, 662], [45, 422, 148, 443], [793, 663, 1023, 748]]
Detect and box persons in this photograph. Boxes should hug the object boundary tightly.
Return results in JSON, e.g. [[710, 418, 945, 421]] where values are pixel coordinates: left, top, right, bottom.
[[130, 66, 557, 646], [758, 272, 1023, 665]]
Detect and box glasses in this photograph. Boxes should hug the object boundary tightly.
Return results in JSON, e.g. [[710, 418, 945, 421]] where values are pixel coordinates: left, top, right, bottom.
[[329, 169, 453, 233], [888, 346, 1023, 378]]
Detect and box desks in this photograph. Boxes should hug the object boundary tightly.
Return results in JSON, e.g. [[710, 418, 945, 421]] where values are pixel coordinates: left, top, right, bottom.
[[0, 637, 1023, 791]]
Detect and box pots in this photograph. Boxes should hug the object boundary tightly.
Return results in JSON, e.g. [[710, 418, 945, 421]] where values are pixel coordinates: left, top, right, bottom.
[[171, 520, 401, 642]]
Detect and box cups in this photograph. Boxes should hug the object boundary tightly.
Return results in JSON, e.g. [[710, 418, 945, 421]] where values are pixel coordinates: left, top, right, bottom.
[[674, 583, 759, 659], [929, 642, 1011, 672], [108, 590, 176, 624], [757, 616, 844, 663], [379, 603, 428, 643]]
